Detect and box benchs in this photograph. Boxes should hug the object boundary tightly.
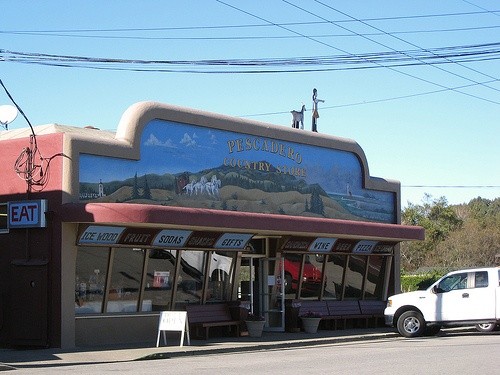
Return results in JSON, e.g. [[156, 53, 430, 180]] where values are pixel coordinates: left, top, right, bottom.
[[186, 306, 243, 339], [298, 300, 385, 330]]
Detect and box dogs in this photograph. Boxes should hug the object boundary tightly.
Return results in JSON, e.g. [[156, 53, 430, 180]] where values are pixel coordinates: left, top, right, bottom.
[[290, 105, 307, 129]]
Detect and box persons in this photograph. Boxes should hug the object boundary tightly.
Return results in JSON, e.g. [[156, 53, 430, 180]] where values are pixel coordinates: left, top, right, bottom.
[[312, 88, 325, 132]]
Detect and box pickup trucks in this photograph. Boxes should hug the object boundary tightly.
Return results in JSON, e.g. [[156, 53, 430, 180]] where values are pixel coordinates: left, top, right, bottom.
[[382, 267, 499, 337]]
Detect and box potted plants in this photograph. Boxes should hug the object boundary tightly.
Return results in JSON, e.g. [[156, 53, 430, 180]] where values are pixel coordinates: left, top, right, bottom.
[[245, 313, 267, 338], [300, 311, 323, 334]]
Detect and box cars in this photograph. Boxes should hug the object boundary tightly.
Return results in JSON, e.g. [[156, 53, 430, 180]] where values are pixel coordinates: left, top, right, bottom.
[[276, 251, 329, 295], [143, 245, 242, 286]]
